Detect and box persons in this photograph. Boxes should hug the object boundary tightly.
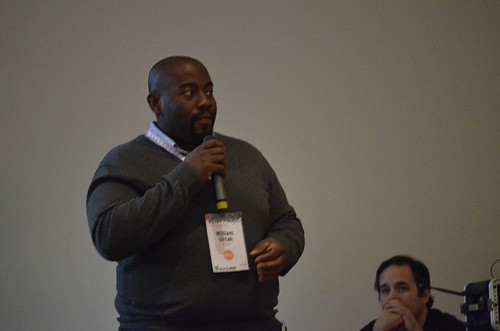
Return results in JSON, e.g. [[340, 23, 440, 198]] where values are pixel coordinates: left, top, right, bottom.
[[358, 255, 466, 331], [85, 56, 305, 331]]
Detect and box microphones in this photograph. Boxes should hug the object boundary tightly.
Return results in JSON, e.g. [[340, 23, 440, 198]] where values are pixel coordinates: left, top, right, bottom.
[[204, 135, 228, 210]]
